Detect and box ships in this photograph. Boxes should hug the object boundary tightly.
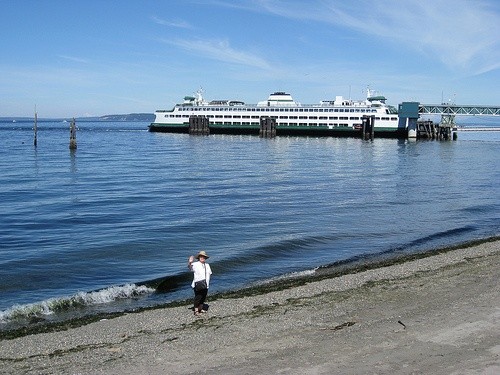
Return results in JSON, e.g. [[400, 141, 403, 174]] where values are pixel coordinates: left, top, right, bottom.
[[148, 88, 420, 138]]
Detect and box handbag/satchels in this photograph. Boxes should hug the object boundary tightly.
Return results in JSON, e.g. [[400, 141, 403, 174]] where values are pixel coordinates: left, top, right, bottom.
[[195, 279, 207, 292]]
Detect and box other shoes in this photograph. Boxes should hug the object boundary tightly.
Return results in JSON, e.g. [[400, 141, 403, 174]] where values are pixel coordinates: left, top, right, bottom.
[[194, 309, 207, 316]]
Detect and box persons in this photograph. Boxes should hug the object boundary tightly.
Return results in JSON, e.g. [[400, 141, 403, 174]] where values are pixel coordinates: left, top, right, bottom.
[[188, 250, 212, 316]]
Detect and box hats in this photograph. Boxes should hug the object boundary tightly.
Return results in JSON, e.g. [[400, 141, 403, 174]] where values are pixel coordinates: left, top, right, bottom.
[[196, 251, 210, 259]]
